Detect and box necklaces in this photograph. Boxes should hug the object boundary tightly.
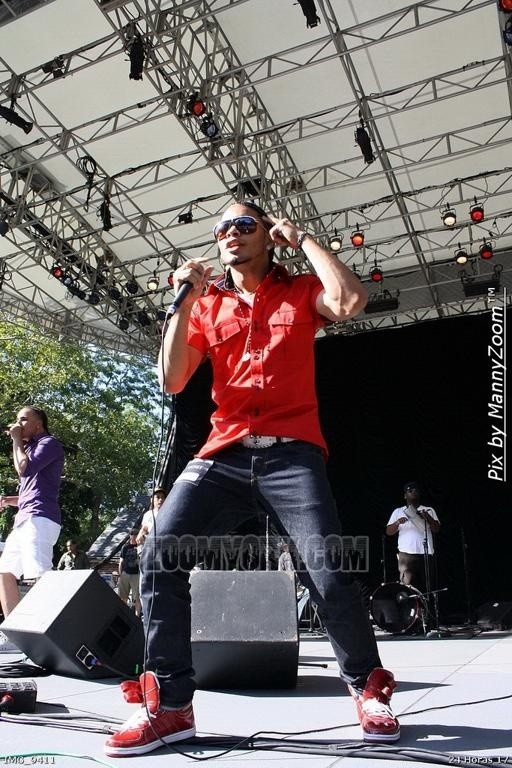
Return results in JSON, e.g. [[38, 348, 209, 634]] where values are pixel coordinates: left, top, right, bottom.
[[230, 285, 257, 361]]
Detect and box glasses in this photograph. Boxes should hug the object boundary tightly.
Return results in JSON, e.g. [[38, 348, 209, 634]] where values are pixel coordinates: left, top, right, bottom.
[[213, 215, 269, 240]]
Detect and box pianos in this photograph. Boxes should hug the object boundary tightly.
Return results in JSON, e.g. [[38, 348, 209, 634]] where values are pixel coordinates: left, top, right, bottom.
[[25, 572, 115, 584]]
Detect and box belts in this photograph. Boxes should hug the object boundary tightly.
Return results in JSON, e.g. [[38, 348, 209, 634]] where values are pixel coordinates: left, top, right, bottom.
[[234, 434, 298, 449]]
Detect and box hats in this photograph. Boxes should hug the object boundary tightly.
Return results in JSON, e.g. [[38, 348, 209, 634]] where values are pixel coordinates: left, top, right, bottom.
[[403, 481, 417, 494]]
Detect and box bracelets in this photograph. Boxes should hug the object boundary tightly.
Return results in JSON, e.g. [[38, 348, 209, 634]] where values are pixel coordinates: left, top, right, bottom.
[[296, 229, 308, 250]]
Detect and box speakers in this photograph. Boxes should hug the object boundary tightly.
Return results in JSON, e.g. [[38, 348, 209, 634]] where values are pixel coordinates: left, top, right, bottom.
[[1, 569, 147, 681], [190, 571, 299, 693]]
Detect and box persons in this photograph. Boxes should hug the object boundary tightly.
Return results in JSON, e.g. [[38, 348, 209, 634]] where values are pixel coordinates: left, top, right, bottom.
[[135, 487, 169, 605], [278, 535, 314, 619], [385, 481, 442, 634], [57, 538, 91, 570], [117, 528, 144, 618], [1, 405, 69, 654], [103, 196, 403, 760]]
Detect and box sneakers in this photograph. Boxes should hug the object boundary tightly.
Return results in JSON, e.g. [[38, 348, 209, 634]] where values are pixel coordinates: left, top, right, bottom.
[[0, 631, 23, 654], [104, 671, 197, 757], [347, 667, 400, 744]]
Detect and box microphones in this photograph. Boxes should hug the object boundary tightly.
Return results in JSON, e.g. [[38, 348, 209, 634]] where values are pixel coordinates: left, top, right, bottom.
[[417, 509, 431, 513], [164, 263, 204, 323]]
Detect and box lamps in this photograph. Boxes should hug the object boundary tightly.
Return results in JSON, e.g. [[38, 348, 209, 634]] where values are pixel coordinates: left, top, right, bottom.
[[298, 0, 321, 28], [330, 208, 382, 282], [354, 118, 376, 164], [50, 257, 177, 330], [0, 103, 33, 134], [440, 175, 499, 265], [129, 34, 144, 81], [185, 92, 206, 117], [200, 112, 218, 138], [100, 202, 113, 231], [496, 0, 512, 47]]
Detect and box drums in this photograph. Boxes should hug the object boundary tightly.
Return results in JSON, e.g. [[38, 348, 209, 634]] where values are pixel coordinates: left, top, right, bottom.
[[371, 582, 429, 633]]
